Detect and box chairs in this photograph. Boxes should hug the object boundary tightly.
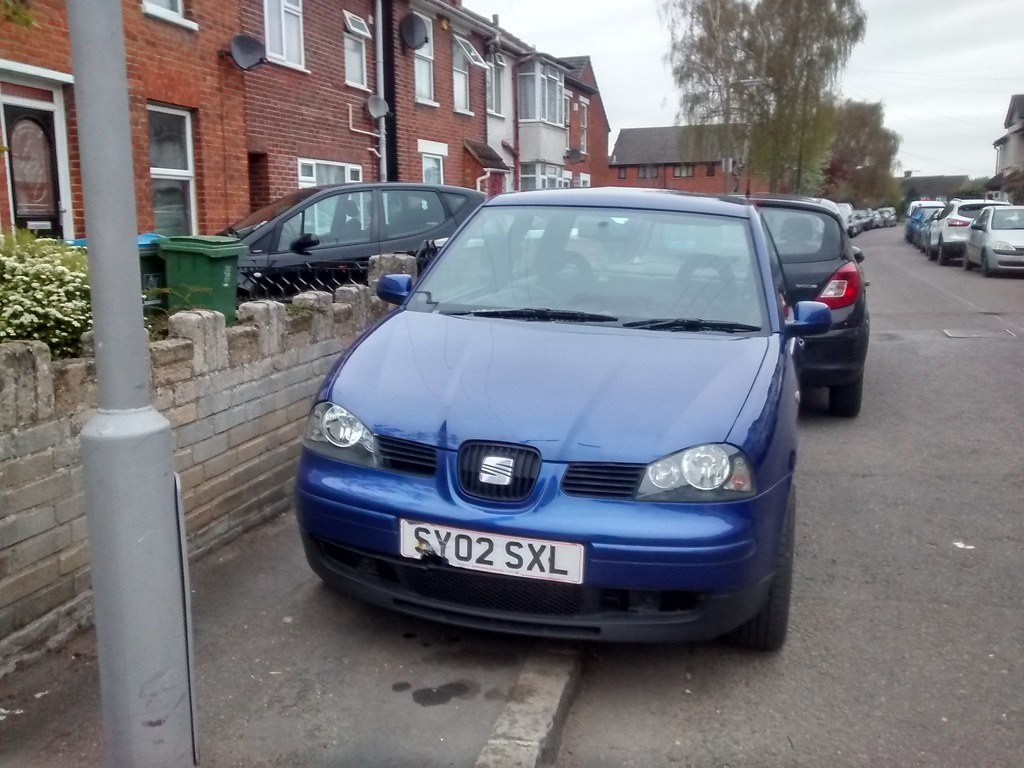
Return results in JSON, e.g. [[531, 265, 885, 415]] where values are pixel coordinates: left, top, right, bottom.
[[778, 217, 818, 254], [675, 253, 736, 308], [340, 200, 361, 241], [540, 250, 597, 303]]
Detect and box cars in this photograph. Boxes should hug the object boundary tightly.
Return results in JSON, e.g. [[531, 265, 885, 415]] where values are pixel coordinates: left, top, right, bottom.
[[834, 199, 947, 261], [960, 204, 1024, 277], [289, 186, 830, 655], [622, 190, 872, 421]]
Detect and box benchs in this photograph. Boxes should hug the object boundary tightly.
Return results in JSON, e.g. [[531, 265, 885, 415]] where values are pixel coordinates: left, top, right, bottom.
[[386, 208, 429, 238]]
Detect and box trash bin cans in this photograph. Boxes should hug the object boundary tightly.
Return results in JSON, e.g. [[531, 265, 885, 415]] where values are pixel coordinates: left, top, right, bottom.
[[151, 233, 250, 327], [70, 234, 168, 342]]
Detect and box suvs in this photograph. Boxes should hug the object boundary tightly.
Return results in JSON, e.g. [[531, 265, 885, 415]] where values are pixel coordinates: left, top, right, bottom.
[[212, 178, 490, 305], [923, 198, 1016, 265]]
